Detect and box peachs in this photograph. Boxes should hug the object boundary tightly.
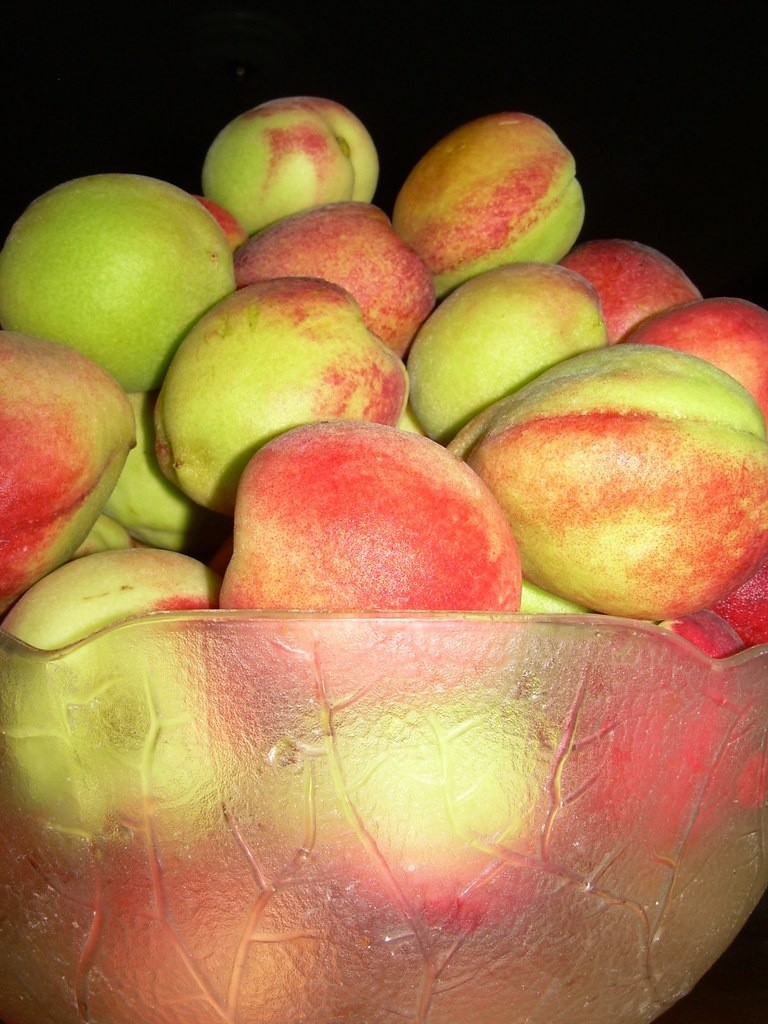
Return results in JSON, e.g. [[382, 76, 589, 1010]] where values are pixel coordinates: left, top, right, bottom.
[[0, 95, 768, 1024]]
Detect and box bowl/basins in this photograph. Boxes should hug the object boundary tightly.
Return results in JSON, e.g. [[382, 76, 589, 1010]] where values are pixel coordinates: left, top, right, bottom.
[[0, 614, 768, 1024]]
